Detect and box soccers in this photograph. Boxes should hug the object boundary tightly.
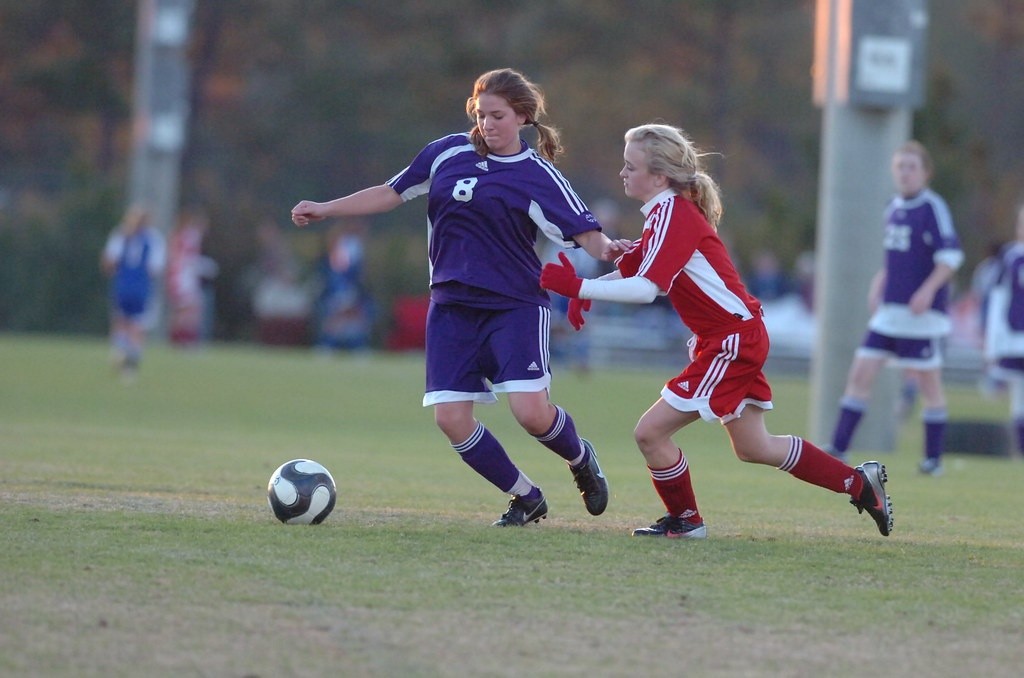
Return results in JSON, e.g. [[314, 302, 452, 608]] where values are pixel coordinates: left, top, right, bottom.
[[267, 458, 337, 525]]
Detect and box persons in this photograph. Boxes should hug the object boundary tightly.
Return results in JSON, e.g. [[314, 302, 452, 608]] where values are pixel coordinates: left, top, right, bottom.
[[807, 143, 1024, 479], [291, 69, 635, 526], [99, 202, 377, 377], [539, 124, 893, 538], [739, 242, 812, 316]]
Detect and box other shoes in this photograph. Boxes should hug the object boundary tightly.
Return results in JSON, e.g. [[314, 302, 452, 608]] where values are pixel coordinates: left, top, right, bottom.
[[918, 458, 944, 476], [820, 442, 850, 463]]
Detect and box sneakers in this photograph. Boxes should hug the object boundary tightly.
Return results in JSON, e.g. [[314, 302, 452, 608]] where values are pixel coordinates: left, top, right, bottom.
[[567, 439, 609, 516], [492, 487, 547, 527], [630, 512, 707, 539], [850, 461, 894, 536]]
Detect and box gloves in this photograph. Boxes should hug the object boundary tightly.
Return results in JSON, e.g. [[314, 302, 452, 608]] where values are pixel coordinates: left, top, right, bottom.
[[569, 297, 592, 331], [542, 252, 584, 301]]
